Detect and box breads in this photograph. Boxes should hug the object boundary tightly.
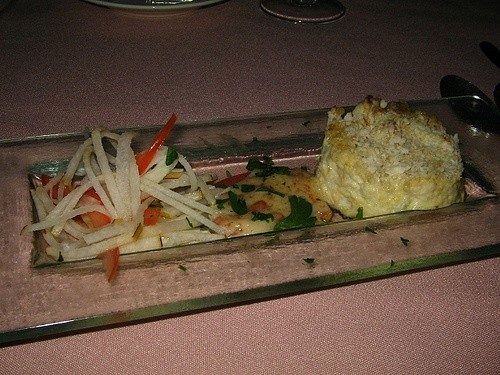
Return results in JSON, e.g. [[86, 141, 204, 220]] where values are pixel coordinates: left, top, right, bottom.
[[317, 95, 462, 220]]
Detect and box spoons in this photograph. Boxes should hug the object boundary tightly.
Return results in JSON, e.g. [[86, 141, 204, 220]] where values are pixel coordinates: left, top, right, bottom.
[[440, 73, 500, 136]]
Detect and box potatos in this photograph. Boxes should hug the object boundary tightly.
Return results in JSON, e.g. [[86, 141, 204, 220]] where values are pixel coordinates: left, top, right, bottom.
[[19, 125, 230, 262]]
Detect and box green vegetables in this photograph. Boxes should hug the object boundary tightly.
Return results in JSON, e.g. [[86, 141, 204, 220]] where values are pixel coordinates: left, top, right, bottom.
[[164, 146, 318, 232]]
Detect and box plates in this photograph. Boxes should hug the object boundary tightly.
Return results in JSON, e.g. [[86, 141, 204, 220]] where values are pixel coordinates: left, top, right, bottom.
[[88, 0, 225, 16]]
